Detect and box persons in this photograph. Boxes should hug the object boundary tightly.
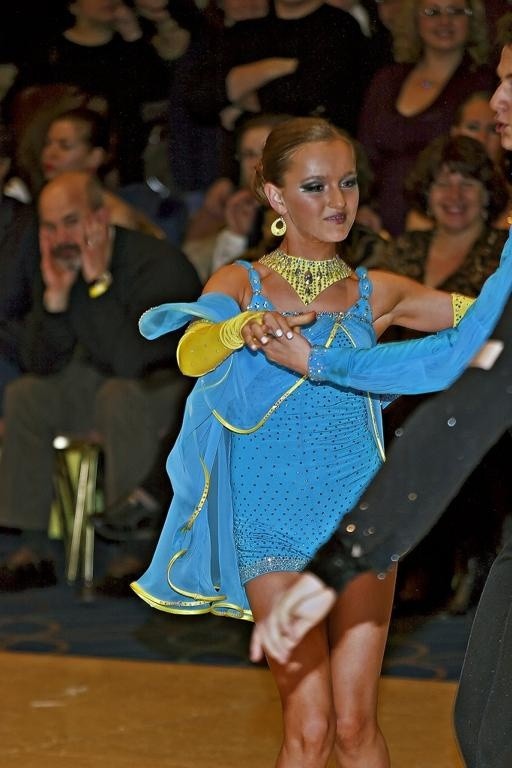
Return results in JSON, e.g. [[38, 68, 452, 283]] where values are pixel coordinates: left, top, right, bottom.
[[246, 232, 512, 768], [174, 106, 512, 768]]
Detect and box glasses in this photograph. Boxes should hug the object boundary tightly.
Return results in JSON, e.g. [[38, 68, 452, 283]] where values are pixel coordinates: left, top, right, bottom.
[[418, 4, 472, 17]]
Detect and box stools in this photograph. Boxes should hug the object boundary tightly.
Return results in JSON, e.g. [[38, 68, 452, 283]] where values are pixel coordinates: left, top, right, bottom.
[[47, 433, 108, 601]]
